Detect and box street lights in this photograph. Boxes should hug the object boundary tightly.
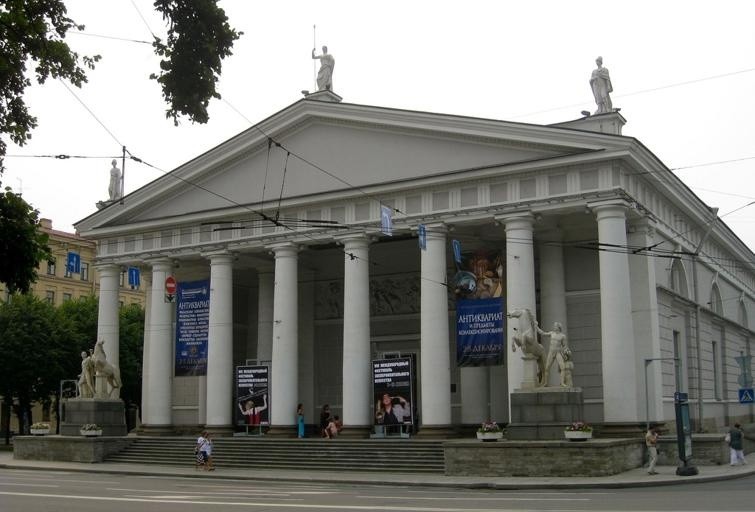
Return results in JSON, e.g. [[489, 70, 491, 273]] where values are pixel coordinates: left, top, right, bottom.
[[643, 355, 682, 435]]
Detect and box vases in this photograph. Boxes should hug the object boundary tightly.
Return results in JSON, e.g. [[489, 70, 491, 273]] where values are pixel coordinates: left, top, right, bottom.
[[30, 429, 50, 436], [477, 433, 503, 442], [80, 431, 102, 437], [564, 432, 592, 442]]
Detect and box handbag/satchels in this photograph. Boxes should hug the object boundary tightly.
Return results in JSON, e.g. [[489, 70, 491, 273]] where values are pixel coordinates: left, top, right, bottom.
[[194, 447, 199, 454]]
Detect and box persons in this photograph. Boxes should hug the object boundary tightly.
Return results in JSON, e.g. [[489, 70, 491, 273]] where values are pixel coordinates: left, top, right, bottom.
[[589, 54, 616, 113], [76, 352, 98, 399], [375, 392, 409, 425], [310, 44, 336, 92], [725, 422, 747, 467], [296, 403, 305, 439], [108, 159, 124, 199], [645, 425, 659, 476], [536, 321, 574, 387], [237, 394, 267, 424], [319, 404, 343, 440], [193, 431, 216, 472]]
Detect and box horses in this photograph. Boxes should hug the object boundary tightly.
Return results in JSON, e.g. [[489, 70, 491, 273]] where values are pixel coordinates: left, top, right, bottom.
[[90, 339, 122, 397], [508, 308, 547, 384]]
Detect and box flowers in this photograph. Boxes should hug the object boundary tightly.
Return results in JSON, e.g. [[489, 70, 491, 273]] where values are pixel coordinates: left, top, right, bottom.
[[80, 424, 102, 430], [478, 421, 503, 432], [30, 424, 50, 429], [565, 422, 592, 432]]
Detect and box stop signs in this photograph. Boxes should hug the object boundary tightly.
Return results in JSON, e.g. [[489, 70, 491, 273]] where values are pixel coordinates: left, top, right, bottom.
[[165, 276, 176, 293]]
